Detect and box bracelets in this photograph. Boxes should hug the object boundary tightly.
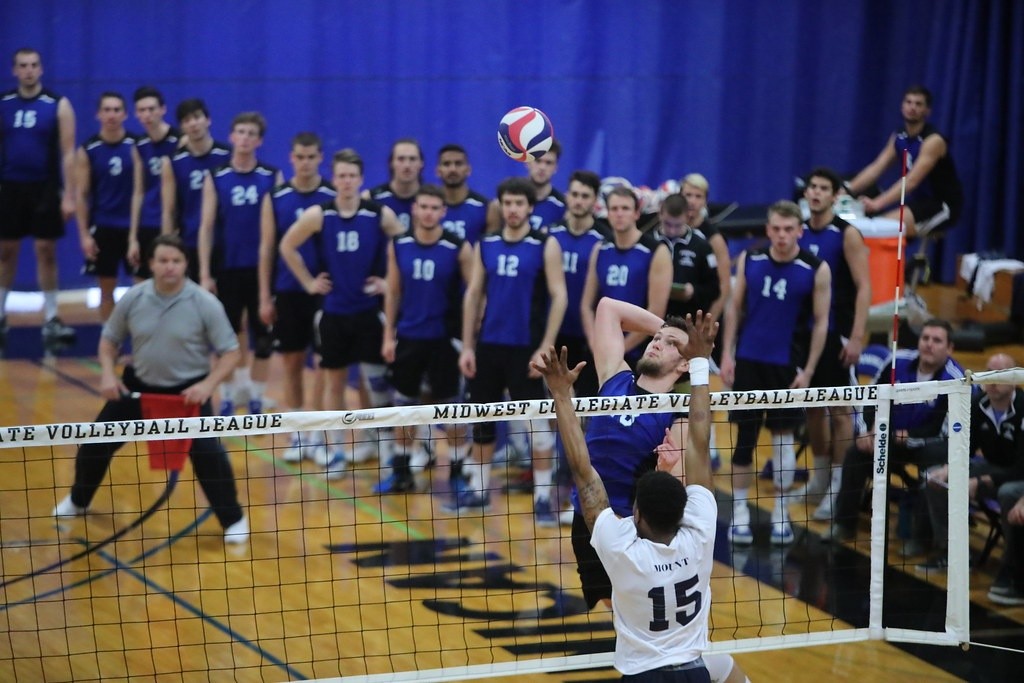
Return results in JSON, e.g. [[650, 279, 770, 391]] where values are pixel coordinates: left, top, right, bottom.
[[687, 356, 709, 385]]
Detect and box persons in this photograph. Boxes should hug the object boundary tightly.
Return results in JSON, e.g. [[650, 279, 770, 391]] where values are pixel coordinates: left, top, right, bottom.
[[0, 48, 77, 337], [529, 309, 719, 683], [570, 296, 751, 683], [53, 236, 250, 544], [75, 86, 1024, 604]]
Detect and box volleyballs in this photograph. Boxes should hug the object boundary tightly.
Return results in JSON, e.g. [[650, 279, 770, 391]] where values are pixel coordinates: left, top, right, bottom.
[[496, 105, 555, 162]]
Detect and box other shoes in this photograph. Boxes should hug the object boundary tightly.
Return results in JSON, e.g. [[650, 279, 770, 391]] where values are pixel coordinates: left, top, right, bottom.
[[42, 316, 75, 336], [789, 480, 827, 502], [326, 452, 345, 479], [446, 461, 466, 495], [304, 441, 335, 465], [283, 439, 305, 460], [990, 576, 1011, 594], [372, 456, 417, 494], [443, 488, 492, 515], [249, 401, 261, 414], [728, 507, 753, 543], [916, 549, 975, 574], [53, 495, 87, 517], [813, 486, 839, 518], [770, 510, 794, 543], [218, 401, 232, 417], [986, 586, 1024, 605], [534, 497, 557, 526], [224, 517, 249, 542]]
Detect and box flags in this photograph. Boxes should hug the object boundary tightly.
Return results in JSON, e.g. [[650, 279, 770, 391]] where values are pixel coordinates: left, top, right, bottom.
[[140, 393, 200, 471]]
